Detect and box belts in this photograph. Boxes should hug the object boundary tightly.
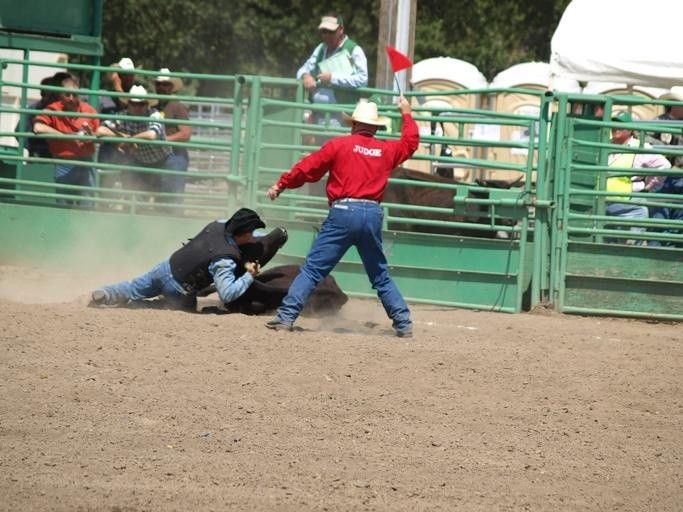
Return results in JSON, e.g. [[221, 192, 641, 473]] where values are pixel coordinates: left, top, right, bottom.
[[330, 197, 383, 204]]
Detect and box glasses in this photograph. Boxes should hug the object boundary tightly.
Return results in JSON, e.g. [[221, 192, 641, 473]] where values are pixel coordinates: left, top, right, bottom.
[[130, 102, 145, 106]]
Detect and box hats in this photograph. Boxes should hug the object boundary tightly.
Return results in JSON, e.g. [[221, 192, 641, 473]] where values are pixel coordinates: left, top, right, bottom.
[[341, 98, 392, 126], [318, 12, 344, 32], [611, 111, 632, 122], [659, 86, 683, 100], [149, 68, 183, 92], [109, 57, 134, 71], [118, 85, 159, 107], [224, 208, 266, 234]]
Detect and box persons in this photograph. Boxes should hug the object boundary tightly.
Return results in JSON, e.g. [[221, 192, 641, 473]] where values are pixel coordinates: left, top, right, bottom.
[[263, 95, 421, 337], [87, 208, 267, 311], [606, 86, 683, 247], [15, 57, 192, 215], [296, 12, 368, 144]]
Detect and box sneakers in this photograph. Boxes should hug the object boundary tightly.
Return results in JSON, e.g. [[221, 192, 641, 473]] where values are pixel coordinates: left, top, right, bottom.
[[264, 321, 293, 333], [86, 290, 105, 307], [395, 330, 414, 338]]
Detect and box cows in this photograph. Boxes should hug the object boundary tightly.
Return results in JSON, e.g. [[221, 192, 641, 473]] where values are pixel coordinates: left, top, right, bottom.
[[179, 226, 349, 320], [381, 167, 524, 239]]
